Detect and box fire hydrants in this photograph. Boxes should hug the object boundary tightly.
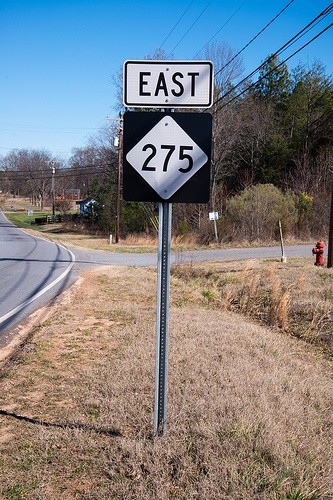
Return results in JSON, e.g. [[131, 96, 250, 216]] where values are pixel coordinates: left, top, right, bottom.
[[312, 241, 325, 266]]
